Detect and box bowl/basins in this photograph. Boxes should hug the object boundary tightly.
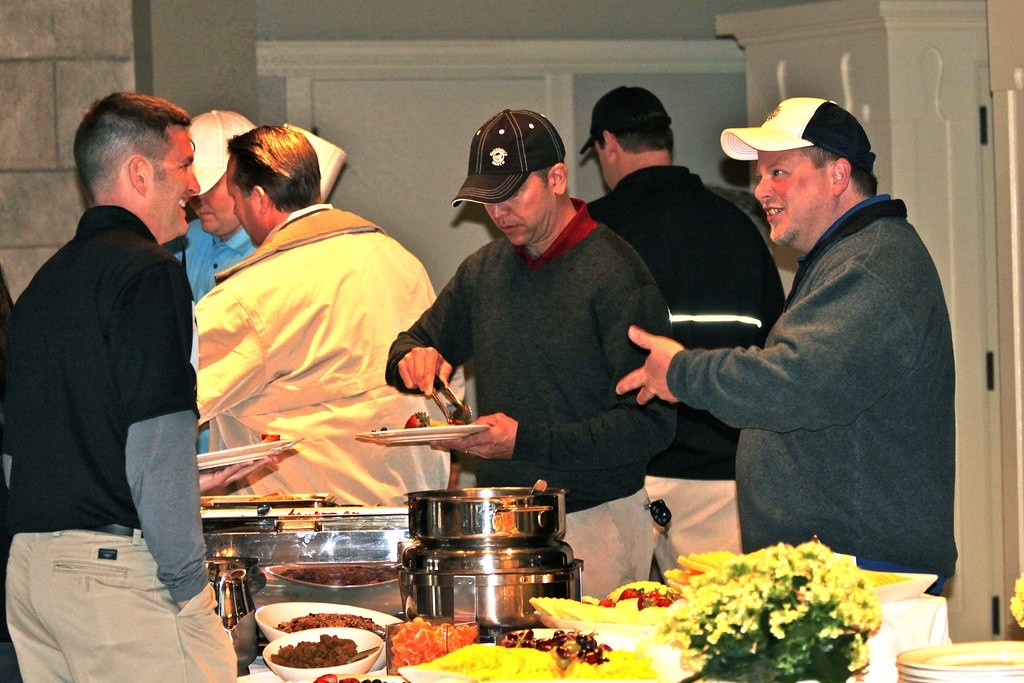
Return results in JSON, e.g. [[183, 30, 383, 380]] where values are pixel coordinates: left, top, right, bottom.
[[262, 627, 383, 681], [255, 602, 404, 672]]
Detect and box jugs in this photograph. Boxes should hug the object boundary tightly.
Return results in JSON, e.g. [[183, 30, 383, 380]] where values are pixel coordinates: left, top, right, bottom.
[[213, 576, 255, 668]]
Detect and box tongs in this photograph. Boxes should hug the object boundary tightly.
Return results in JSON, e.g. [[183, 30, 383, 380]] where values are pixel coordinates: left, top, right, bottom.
[[432, 374, 472, 424]]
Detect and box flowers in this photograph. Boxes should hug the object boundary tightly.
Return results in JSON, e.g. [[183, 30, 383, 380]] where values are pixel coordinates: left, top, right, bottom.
[[657, 539, 883, 683]]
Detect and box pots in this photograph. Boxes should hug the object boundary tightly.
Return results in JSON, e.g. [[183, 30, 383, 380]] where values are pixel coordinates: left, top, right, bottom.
[[396, 487, 584, 643]]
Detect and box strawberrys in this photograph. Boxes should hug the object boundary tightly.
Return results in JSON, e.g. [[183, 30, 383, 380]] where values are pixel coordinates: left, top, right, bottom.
[[405, 411, 429, 429], [313, 673, 361, 683], [598, 589, 679, 610]]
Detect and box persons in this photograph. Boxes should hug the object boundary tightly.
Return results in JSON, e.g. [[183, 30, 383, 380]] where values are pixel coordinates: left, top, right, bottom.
[[0, 85, 245, 683], [178, 123, 449, 510], [380, 106, 681, 610], [567, 84, 788, 582], [1, 256, 38, 680], [138, 107, 286, 454], [605, 91, 960, 600]]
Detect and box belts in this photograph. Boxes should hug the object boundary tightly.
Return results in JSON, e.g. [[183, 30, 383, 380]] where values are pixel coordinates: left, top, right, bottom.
[[88, 524, 143, 537]]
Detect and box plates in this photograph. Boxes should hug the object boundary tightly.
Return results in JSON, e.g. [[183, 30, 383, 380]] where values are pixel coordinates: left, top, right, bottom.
[[196, 435, 305, 470], [398, 628, 694, 683], [896, 641, 1024, 683], [877, 573, 939, 604], [533, 608, 666, 635], [354, 425, 489, 444]]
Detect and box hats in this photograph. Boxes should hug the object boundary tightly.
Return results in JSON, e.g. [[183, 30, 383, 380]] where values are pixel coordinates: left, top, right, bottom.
[[451, 108, 568, 207], [581, 84, 672, 153], [284, 124, 346, 204], [188, 110, 259, 197], [719, 96, 879, 168]]
